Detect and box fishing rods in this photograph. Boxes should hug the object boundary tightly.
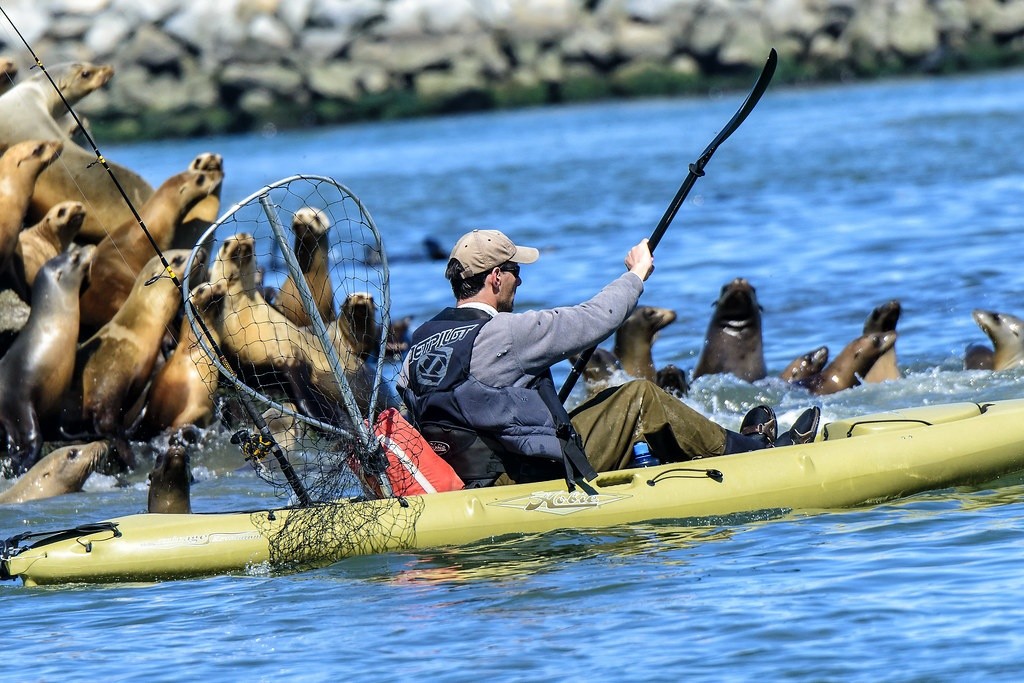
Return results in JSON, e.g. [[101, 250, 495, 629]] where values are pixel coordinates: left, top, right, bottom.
[[0, 7, 314, 508]]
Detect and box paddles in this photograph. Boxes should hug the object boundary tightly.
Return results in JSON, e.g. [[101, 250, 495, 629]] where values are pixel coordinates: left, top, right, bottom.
[[556, 47, 777, 406]]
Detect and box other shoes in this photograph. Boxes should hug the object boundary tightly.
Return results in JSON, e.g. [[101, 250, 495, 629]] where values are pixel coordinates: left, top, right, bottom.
[[723, 406, 821, 455], [740, 405, 778, 445]]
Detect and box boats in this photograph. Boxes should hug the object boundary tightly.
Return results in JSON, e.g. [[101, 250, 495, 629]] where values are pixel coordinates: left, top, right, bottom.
[[2, 385, 1024, 590]]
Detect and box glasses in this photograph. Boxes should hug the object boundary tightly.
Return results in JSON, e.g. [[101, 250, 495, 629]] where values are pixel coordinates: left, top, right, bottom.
[[483, 266, 521, 278]]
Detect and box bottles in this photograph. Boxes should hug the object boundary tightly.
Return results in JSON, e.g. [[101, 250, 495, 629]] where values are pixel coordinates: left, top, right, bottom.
[[633, 441, 659, 469]]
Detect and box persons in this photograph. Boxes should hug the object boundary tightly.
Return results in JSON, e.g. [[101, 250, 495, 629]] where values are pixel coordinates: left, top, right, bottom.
[[387, 230, 825, 490]]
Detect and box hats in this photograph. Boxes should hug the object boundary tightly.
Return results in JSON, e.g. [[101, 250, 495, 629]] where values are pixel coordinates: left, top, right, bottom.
[[447, 229, 539, 279]]
